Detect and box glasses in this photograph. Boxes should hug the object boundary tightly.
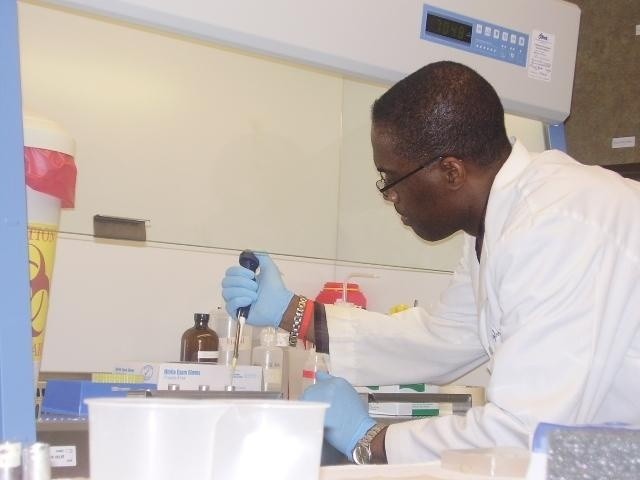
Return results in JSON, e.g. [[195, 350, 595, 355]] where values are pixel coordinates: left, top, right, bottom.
[[375, 155, 463, 198]]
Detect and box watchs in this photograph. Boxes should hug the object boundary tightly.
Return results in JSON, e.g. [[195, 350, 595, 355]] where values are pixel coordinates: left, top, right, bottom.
[[351, 422, 387, 464]]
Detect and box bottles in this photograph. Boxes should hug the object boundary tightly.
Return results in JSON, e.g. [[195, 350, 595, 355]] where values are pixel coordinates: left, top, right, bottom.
[[182, 312, 331, 401]]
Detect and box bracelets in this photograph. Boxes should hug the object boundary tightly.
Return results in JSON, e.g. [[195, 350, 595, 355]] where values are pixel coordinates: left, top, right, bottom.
[[287, 294, 306, 348], [298, 299, 314, 342]]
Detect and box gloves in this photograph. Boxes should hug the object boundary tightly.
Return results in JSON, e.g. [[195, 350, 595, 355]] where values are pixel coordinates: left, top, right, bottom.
[[222, 252, 295, 328], [303, 371, 378, 459]]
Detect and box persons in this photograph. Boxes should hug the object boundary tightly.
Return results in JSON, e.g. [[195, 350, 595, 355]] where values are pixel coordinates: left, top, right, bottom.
[[220, 60, 639, 464]]
